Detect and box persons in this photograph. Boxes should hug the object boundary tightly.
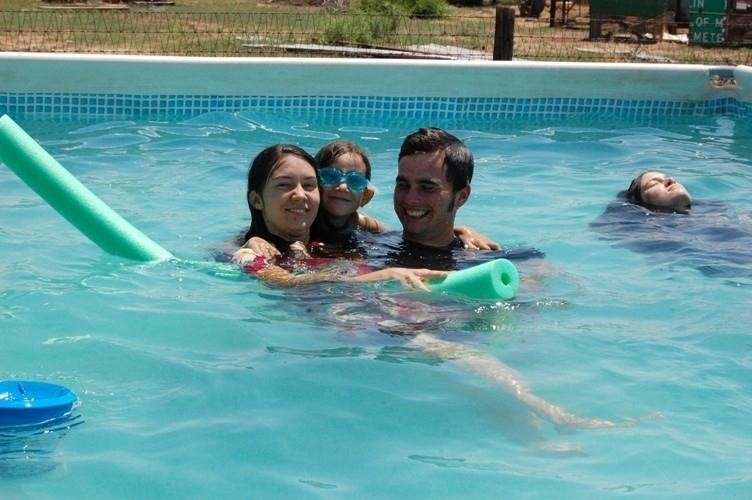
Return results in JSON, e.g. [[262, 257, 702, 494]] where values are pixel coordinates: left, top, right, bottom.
[[243, 125, 498, 255], [583, 168, 752, 282], [240, 143, 322, 251], [312, 141, 383, 231]]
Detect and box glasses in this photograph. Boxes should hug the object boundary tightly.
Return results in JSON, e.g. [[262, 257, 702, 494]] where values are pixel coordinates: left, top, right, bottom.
[[316, 165, 371, 193]]
